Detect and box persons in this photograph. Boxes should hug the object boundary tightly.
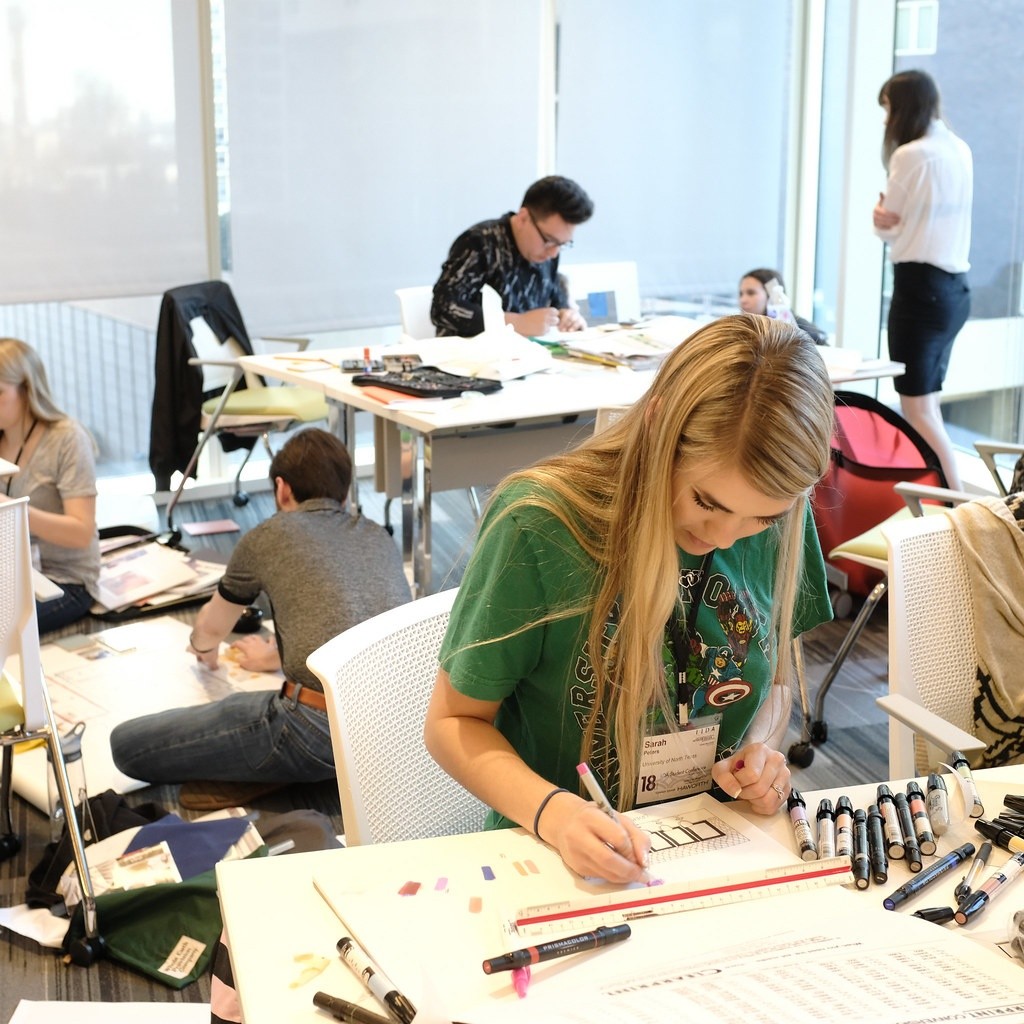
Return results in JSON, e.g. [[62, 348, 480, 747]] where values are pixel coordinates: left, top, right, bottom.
[[872, 69, 973, 508], [428, 176, 594, 335], [110, 429, 414, 811], [422, 313, 835, 886], [0, 338, 100, 634], [737, 268, 828, 346]]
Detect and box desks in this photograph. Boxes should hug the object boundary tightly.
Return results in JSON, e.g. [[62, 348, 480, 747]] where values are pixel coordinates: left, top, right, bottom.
[[213, 766, 1024, 1024], [237, 320, 906, 600]]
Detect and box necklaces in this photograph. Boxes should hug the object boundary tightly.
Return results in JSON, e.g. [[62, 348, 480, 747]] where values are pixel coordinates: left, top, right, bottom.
[[7, 420, 40, 496], [677, 555, 706, 588]]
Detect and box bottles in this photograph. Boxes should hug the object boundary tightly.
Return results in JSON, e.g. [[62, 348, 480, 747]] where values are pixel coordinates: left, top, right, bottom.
[[44, 722, 86, 848], [766, 286, 791, 325]]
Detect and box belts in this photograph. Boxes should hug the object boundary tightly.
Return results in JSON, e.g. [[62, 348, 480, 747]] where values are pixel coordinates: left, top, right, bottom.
[[284, 681, 327, 710]]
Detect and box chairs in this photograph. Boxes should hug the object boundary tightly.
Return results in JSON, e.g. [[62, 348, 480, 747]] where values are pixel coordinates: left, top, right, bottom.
[[163, 282, 328, 540], [0, 496, 111, 968], [875, 515, 1024, 775], [812, 439, 1024, 741], [382, 286, 489, 534], [304, 588, 492, 845]]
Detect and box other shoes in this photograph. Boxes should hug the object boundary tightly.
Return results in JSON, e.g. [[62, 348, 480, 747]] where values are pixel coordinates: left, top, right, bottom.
[[177, 778, 270, 810]]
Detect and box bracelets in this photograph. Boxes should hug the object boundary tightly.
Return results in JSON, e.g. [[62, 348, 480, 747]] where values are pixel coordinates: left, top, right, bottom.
[[534, 788, 570, 840], [190, 632, 213, 653]]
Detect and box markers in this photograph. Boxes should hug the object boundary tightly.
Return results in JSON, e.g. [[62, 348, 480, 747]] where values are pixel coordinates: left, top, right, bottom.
[[835, 796, 853, 863], [926, 773, 949, 835], [816, 799, 834, 859], [883, 843, 975, 910], [483, 923, 631, 975], [954, 851, 1024, 925], [894, 793, 922, 873], [910, 906, 954, 925], [866, 805, 888, 884], [313, 991, 399, 1024], [905, 781, 936, 856], [974, 794, 1024, 852], [336, 937, 416, 1024], [854, 809, 870, 890], [787, 788, 818, 861], [877, 784, 906, 859], [951, 750, 984, 818]]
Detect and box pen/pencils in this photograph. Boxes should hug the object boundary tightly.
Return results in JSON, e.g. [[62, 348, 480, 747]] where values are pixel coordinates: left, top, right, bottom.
[[575, 761, 657, 883], [955, 839, 993, 906]]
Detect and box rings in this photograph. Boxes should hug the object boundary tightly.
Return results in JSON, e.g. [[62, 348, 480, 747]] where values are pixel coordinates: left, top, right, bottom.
[[771, 784, 784, 799]]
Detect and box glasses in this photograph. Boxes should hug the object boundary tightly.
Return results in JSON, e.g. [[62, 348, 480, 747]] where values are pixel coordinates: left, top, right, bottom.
[[528, 212, 574, 249]]
